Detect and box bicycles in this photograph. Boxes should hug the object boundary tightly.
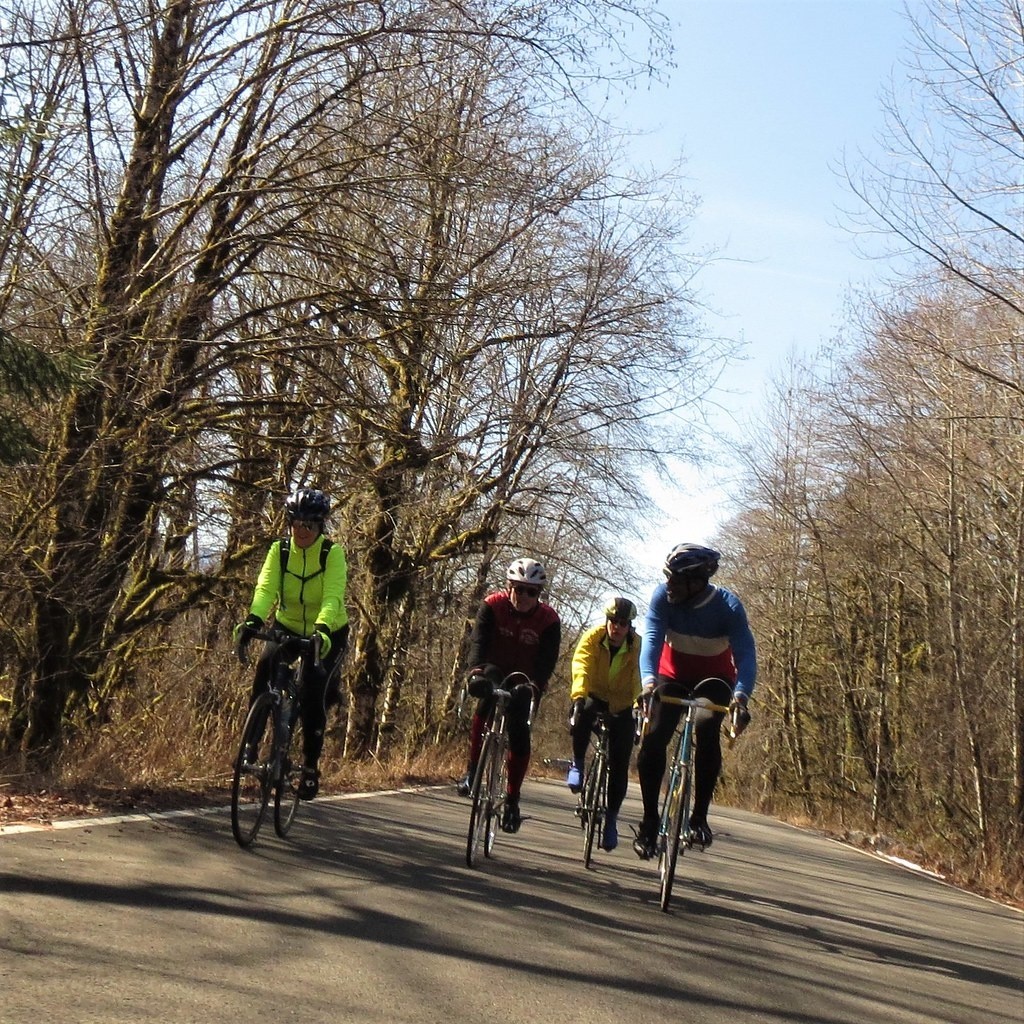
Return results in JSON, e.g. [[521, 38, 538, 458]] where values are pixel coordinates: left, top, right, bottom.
[[569, 695, 644, 870], [446, 669, 538, 869], [633, 676, 747, 912], [229, 624, 323, 848]]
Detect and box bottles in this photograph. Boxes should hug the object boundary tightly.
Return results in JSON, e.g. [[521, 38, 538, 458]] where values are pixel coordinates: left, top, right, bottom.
[[280, 689, 291, 725]]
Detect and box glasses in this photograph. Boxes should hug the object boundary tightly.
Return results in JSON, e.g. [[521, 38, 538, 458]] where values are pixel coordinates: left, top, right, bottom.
[[609, 618, 630, 628], [512, 585, 539, 598], [292, 519, 325, 532], [661, 567, 673, 580]]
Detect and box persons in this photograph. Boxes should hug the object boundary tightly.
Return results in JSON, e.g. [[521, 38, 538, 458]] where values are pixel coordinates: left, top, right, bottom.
[[230, 489, 351, 802], [564, 597, 644, 851], [455, 559, 560, 834], [633, 542, 755, 861]]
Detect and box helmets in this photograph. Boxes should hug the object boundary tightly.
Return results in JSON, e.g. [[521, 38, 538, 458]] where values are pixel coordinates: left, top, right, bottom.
[[604, 598, 637, 620], [285, 489, 329, 517], [665, 543, 721, 580], [506, 558, 546, 585]]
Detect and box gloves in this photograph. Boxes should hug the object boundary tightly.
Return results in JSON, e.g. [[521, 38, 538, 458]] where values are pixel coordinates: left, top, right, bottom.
[[241, 613, 264, 644], [632, 708, 642, 720], [636, 687, 660, 718], [729, 702, 750, 725], [573, 701, 582, 724], [515, 684, 532, 701], [468, 673, 494, 700], [310, 623, 330, 650]]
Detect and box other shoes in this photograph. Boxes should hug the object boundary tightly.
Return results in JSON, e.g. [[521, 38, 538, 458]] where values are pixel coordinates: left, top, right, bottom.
[[502, 805, 520, 834], [604, 828, 617, 848], [299, 765, 318, 801], [458, 773, 473, 795], [245, 744, 257, 764], [690, 818, 713, 845], [643, 818, 660, 845], [567, 766, 582, 788]]
